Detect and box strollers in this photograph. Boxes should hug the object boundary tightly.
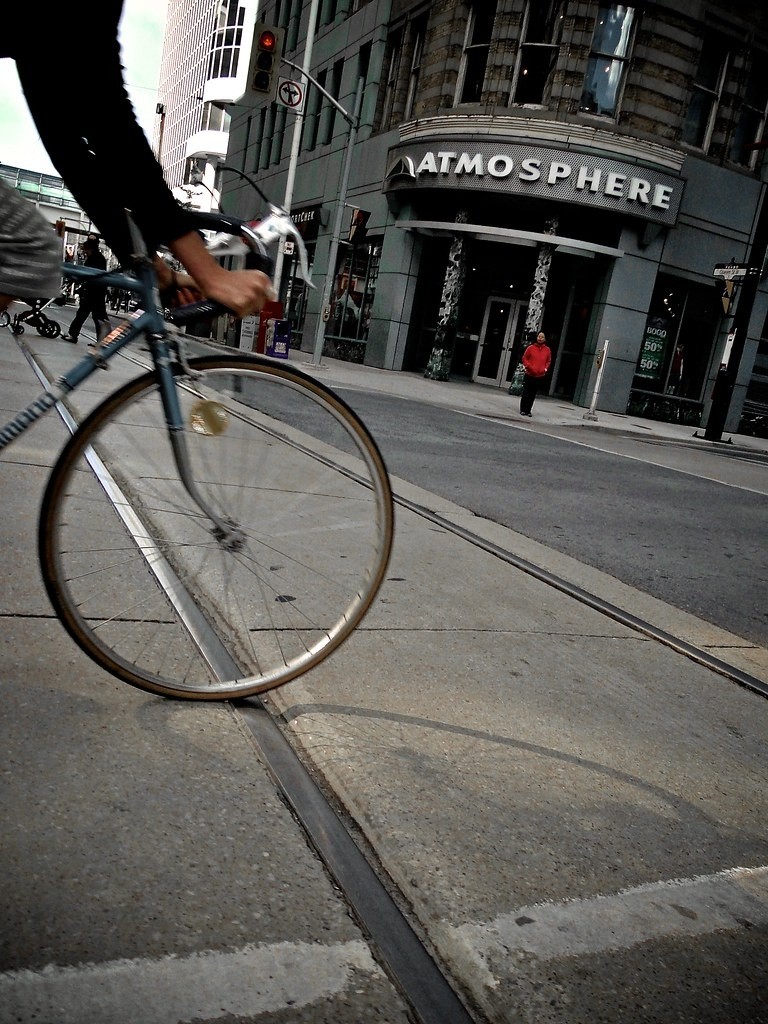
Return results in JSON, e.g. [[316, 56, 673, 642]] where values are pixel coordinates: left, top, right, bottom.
[[10, 282, 74, 339]]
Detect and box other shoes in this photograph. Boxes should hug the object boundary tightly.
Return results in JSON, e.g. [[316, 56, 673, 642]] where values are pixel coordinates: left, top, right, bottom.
[[62, 335, 78, 344], [88, 343, 95, 347], [520, 412, 525, 415], [527, 413, 532, 417]]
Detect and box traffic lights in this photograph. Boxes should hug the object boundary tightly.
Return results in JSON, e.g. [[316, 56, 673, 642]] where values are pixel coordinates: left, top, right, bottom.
[[245, 21, 285, 102], [349, 209, 371, 245], [714, 278, 734, 314], [55, 219, 65, 241]]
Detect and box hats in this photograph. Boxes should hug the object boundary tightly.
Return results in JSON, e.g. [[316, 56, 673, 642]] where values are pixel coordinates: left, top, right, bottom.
[[83, 240, 99, 250]]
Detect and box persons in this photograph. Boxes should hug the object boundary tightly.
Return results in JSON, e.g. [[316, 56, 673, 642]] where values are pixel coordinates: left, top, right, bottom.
[[62, 238, 111, 348], [106, 260, 176, 313], [0, 0, 275, 316], [520, 331, 551, 417]]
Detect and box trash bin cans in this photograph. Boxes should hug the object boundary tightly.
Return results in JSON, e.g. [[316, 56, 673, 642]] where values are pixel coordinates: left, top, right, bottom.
[[263, 318, 292, 358], [226, 318, 241, 348], [239, 315, 260, 352]]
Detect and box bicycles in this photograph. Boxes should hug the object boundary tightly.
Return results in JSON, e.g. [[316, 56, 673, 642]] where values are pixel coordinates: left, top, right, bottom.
[[0, 153, 394, 703]]
